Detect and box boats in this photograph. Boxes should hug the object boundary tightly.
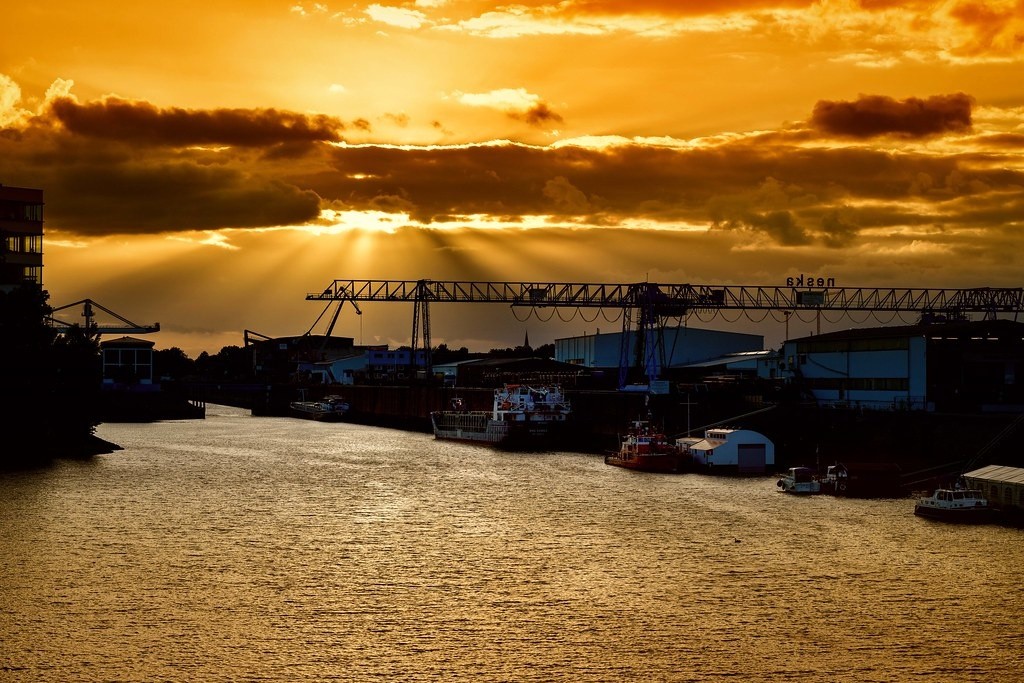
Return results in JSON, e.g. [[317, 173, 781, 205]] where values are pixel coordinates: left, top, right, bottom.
[[778, 465, 822, 494], [289, 395, 353, 421], [603, 413, 686, 473], [913, 482, 1001, 522], [429, 383, 576, 450]]
